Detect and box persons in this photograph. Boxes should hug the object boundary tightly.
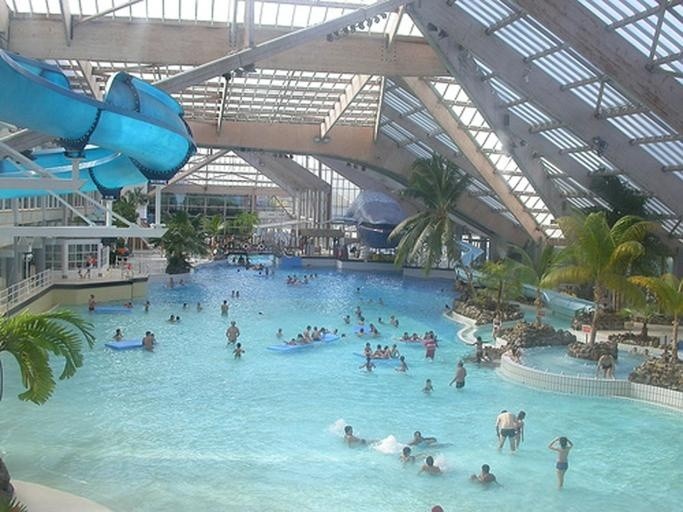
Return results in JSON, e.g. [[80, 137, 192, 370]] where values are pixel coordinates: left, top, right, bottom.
[[340, 409, 524, 488], [126, 301, 151, 311], [465, 336, 491, 361], [88, 295, 95, 312], [344, 306, 399, 337], [449, 361, 466, 389], [422, 380, 432, 396], [220, 290, 239, 316], [277, 326, 345, 346], [593, 351, 615, 380], [122, 258, 129, 279], [363, 330, 439, 370], [226, 321, 245, 358], [490, 312, 501, 341], [639, 349, 648, 356], [168, 302, 200, 321], [83, 255, 94, 280], [287, 273, 317, 284], [167, 277, 183, 288], [629, 347, 637, 354], [546, 436, 574, 492], [233, 254, 269, 274], [113, 329, 155, 350]]
